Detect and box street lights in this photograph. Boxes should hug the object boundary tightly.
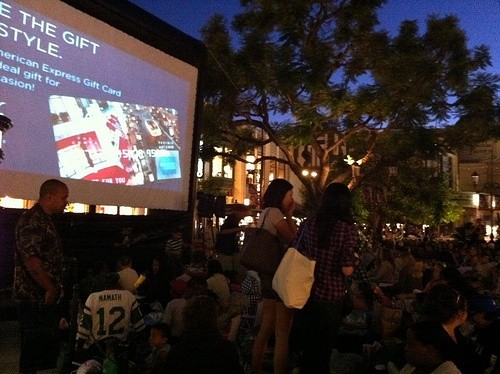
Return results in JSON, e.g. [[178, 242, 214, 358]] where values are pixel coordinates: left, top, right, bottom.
[[473, 170, 500, 242]]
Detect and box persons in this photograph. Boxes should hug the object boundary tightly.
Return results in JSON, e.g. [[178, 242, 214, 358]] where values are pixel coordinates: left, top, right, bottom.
[[12, 179, 69, 374], [334, 222, 500, 374], [289, 182, 359, 374], [251, 179, 297, 374], [59, 226, 260, 374], [215, 206, 247, 282]]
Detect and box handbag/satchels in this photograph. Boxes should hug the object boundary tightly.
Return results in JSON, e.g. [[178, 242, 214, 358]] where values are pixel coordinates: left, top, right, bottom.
[[241, 227, 280, 275], [272, 248, 316, 309]]
